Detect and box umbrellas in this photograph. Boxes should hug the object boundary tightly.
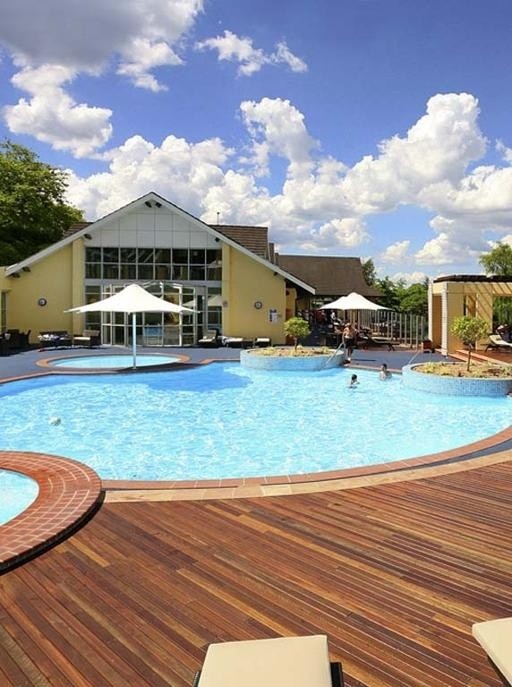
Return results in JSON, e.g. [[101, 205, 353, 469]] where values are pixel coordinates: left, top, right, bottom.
[[62, 283, 201, 366], [320, 291, 393, 321]]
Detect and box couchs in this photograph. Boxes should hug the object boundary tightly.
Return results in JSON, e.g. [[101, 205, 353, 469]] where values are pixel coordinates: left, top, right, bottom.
[[39, 329, 100, 349]]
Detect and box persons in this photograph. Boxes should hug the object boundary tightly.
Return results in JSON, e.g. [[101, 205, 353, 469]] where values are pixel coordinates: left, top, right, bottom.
[[378, 363, 393, 381], [349, 374, 360, 388], [342, 323, 357, 363]]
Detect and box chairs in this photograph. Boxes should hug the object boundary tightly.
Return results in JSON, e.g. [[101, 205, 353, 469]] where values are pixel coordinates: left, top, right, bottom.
[[198, 329, 271, 348], [481, 333, 512, 351], [364, 332, 400, 351]]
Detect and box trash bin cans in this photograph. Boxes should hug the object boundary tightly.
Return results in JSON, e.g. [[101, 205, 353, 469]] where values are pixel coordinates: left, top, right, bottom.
[[423, 340, 432, 353]]
